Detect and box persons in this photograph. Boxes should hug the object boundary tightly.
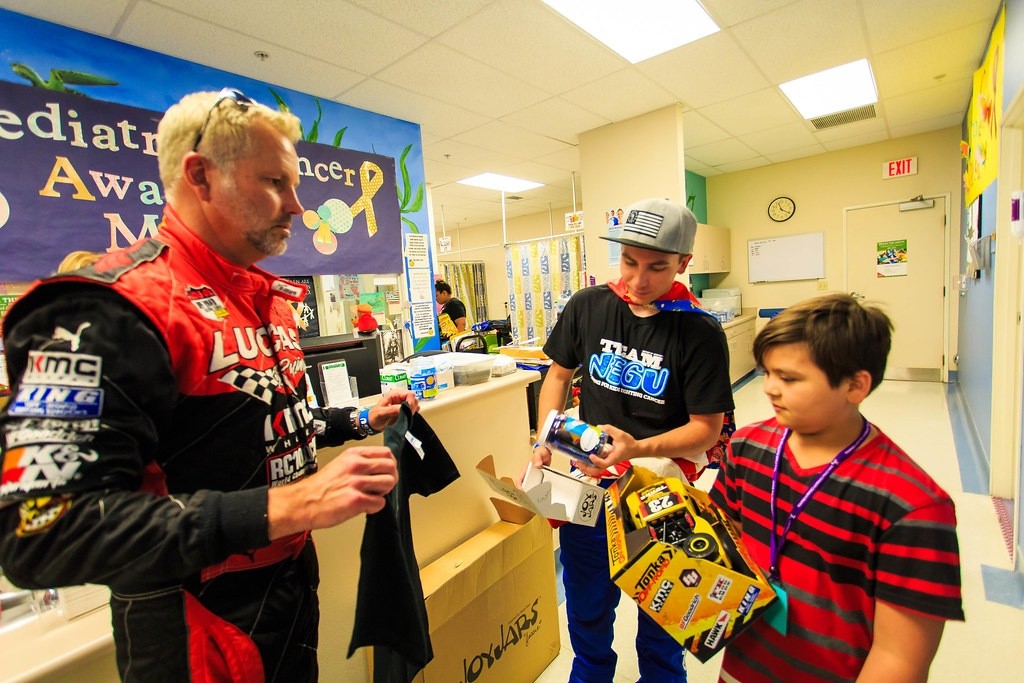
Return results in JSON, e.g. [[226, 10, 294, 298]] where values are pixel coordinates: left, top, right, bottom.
[[435, 280, 466, 349], [0, 87, 420, 683], [516, 197, 736, 683], [709, 292, 967, 683]]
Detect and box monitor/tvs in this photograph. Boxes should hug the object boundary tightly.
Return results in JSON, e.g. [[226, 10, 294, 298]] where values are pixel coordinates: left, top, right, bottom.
[[299, 332, 382, 410]]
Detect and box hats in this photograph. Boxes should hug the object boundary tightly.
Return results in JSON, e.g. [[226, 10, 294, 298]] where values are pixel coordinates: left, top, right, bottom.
[[598, 197, 697, 255]]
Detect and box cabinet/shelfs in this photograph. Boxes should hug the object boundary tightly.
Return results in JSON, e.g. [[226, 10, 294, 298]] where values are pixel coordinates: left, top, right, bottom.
[[722, 318, 756, 386], [301, 331, 382, 406]]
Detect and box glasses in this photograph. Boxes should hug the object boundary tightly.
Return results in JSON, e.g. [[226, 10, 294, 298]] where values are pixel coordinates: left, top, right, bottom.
[[191, 86, 256, 152]]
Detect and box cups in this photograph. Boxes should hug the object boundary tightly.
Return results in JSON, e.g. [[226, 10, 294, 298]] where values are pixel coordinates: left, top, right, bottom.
[[538, 409, 608, 465]]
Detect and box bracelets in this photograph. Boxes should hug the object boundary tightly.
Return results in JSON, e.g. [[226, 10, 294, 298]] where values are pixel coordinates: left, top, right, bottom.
[[533, 442, 552, 455], [349, 407, 370, 437], [359, 410, 380, 435]]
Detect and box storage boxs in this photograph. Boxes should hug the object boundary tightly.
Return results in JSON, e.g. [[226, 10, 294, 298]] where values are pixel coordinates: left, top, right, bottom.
[[437, 352, 493, 385], [702, 287, 742, 316]]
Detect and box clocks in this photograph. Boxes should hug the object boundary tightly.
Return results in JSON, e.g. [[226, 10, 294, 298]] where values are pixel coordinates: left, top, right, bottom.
[[768, 197, 795, 222]]
